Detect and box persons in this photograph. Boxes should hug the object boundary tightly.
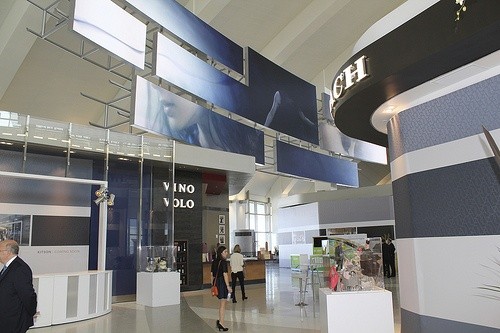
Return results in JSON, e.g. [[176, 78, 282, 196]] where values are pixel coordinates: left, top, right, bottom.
[[210, 246, 232, 331], [0, 240, 37, 333], [382, 238, 396, 278], [230, 244, 248, 303], [147, 82, 262, 163]]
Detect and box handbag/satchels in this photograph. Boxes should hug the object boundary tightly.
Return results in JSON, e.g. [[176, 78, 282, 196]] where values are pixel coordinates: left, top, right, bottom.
[[212, 286, 218, 296]]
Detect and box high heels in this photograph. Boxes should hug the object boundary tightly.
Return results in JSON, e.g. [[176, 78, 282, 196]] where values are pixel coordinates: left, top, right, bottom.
[[216, 320, 229, 331], [242, 296, 248, 301], [232, 298, 237, 303]]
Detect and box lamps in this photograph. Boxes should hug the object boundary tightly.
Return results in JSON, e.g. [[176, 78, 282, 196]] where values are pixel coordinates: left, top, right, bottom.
[[94, 186, 115, 206]]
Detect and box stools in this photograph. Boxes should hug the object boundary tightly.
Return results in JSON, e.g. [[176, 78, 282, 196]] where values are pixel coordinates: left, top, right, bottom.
[[293, 272, 310, 307]]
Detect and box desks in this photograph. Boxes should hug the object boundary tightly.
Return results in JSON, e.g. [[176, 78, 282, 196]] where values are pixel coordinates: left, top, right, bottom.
[[137, 271, 180, 307], [319, 287, 395, 333]]
